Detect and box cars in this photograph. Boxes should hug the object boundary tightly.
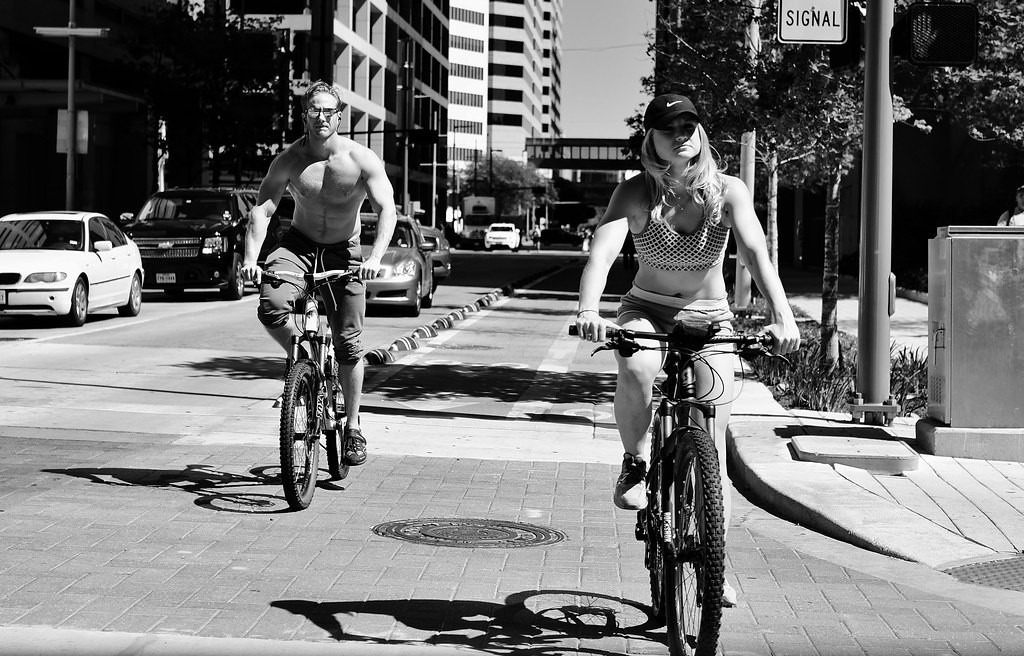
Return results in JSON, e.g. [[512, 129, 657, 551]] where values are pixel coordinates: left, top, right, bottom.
[[295, 214, 438, 318], [534, 228, 585, 247], [415, 224, 453, 280], [483, 222, 521, 252], [0, 210, 146, 327]]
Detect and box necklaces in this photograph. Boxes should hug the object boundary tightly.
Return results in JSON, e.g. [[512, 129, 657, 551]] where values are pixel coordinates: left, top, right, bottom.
[[673, 197, 690, 213]]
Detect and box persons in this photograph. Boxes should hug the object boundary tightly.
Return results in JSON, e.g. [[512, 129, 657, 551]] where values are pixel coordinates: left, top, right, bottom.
[[997, 185, 1024, 227], [240, 82, 397, 464], [576, 93, 801, 610]]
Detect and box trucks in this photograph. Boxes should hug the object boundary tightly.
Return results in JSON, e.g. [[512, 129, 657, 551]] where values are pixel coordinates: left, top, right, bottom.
[[456, 195, 497, 242]]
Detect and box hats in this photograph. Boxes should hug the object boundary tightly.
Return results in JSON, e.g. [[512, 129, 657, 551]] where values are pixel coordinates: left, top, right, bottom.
[[643, 93, 703, 130]]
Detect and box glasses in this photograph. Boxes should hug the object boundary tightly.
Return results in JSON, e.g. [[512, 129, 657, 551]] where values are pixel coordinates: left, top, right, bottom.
[[305, 105, 340, 120]]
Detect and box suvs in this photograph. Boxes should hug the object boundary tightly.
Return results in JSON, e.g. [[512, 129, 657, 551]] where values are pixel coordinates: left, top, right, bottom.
[[118, 186, 281, 302]]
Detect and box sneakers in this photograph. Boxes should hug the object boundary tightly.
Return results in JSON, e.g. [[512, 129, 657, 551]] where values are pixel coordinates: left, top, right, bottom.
[[720, 574, 738, 608], [341, 415, 367, 466], [285, 343, 309, 376], [613, 452, 649, 510]]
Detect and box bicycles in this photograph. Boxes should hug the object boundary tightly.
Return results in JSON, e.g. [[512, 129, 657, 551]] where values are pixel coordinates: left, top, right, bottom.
[[566, 322, 810, 656], [258, 270, 384, 511]]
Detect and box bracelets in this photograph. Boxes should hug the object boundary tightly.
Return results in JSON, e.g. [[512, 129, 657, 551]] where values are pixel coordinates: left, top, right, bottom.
[[576, 307, 600, 317]]
